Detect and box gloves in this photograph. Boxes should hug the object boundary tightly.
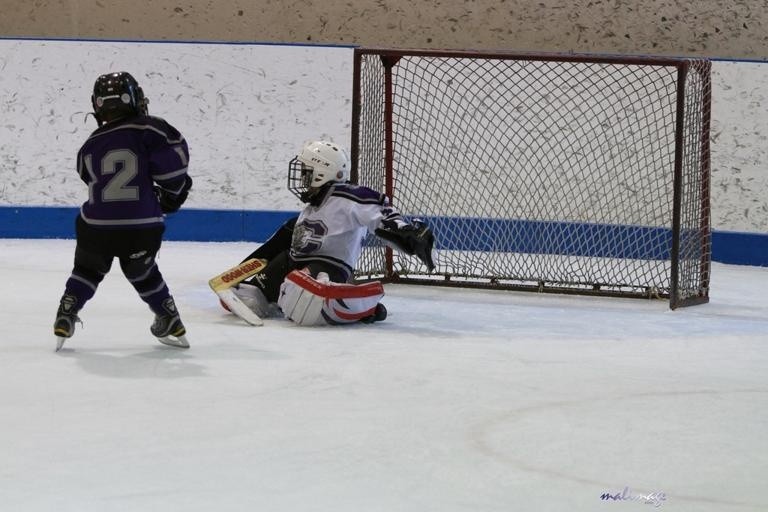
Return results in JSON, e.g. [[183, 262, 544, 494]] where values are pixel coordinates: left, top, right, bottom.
[[157, 174, 192, 213], [401, 217, 436, 273]]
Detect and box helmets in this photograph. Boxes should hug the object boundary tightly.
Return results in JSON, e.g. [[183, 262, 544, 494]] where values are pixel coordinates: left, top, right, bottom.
[[286, 140, 350, 204], [83, 71, 149, 124]]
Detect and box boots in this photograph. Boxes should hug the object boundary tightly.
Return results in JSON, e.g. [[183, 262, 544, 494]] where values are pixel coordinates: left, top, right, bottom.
[[52, 293, 79, 337], [150, 295, 187, 339]]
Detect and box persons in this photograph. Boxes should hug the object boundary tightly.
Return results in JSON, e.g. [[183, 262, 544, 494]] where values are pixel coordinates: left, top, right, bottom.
[[214, 140, 436, 326], [52, 72, 191, 337]]
